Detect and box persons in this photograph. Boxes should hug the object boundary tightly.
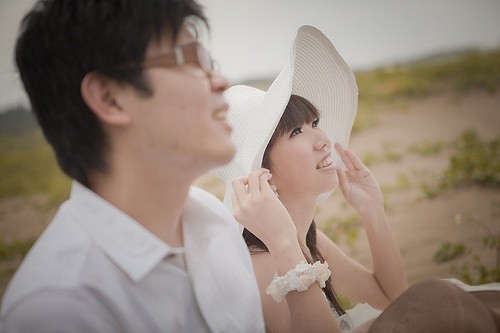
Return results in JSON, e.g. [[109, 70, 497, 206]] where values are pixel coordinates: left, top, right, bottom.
[[0, 0, 267, 333], [208, 24, 406, 333]]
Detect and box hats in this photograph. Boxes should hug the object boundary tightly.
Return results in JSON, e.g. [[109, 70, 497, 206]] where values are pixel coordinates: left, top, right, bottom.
[[210, 24, 359, 240]]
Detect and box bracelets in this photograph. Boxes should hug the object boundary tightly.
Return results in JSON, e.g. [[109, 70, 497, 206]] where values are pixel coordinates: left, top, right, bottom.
[[265, 259, 331, 303]]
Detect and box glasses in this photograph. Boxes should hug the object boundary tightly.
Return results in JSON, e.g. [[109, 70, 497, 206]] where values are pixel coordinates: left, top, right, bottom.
[[101, 39, 214, 77]]
